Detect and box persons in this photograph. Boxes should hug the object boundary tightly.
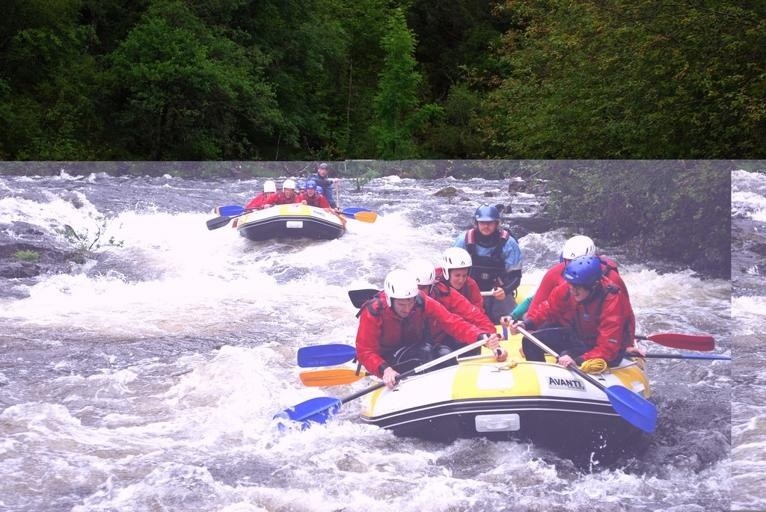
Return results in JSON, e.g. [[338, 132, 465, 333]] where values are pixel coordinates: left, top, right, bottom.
[[449, 205, 524, 326], [354, 234, 637, 389], [242, 161, 340, 214]]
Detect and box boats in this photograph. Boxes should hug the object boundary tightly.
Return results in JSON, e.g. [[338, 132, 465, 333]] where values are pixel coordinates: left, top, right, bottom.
[[239, 202, 345, 238], [362, 347, 655, 436]]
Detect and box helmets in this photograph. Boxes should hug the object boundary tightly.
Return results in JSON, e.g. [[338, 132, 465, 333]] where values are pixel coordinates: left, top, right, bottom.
[[559, 235, 601, 286], [384, 247, 473, 308], [318, 162, 328, 169], [474, 203, 500, 222], [264, 176, 325, 193]]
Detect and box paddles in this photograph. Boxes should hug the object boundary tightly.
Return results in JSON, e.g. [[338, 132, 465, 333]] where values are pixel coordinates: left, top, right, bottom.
[[297, 344, 356, 367], [300, 369, 373, 387], [337, 212, 377, 223], [635, 334, 715, 351], [206, 211, 253, 230], [348, 287, 497, 308], [273, 339, 488, 437], [513, 320, 656, 433], [340, 208, 371, 219], [219, 205, 263, 217]]
